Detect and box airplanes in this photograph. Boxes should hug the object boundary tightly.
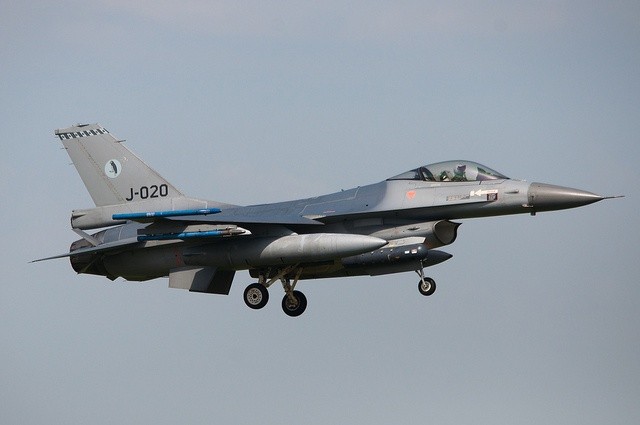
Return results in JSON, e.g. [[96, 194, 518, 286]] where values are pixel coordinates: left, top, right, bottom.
[[28, 121, 627, 317]]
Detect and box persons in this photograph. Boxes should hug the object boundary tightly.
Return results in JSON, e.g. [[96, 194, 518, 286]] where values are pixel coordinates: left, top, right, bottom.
[[451, 163, 467, 182]]
